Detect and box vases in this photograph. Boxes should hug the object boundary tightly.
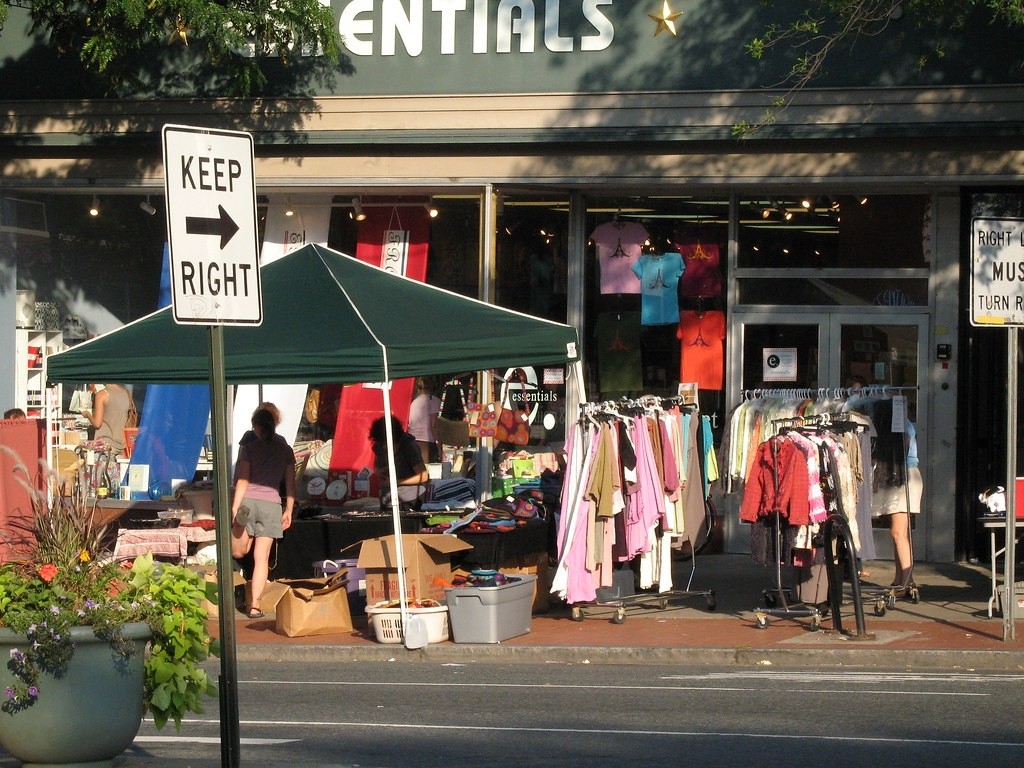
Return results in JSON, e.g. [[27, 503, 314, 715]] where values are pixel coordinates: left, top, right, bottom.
[[0, 622, 154, 768]]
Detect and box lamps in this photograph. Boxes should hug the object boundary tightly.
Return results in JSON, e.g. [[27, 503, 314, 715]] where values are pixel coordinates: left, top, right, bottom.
[[749, 195, 793, 220], [283, 193, 293, 217], [423, 195, 439, 218], [89, 195, 100, 216], [352, 194, 367, 221], [140, 195, 157, 215]]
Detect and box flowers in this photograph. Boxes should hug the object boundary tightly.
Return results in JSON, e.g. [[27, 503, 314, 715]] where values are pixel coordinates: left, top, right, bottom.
[[1, 444, 247, 734]]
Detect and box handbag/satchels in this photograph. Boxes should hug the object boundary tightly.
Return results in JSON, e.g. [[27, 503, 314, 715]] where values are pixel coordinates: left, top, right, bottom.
[[467, 370, 497, 437], [494, 368, 529, 445], [126, 389, 137, 428], [62, 314, 88, 339], [69, 384, 92, 413], [434, 380, 469, 447]]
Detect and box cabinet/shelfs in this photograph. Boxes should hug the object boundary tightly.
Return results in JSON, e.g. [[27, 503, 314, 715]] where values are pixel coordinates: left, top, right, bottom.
[[15, 328, 64, 419]]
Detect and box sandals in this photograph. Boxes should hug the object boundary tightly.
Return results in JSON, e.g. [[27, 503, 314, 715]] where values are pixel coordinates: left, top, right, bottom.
[[235, 505, 250, 527], [249, 607, 265, 619]]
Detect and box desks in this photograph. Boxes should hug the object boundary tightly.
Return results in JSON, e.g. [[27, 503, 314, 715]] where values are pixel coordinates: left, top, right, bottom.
[[984, 522, 1024, 618], [290, 505, 465, 560]]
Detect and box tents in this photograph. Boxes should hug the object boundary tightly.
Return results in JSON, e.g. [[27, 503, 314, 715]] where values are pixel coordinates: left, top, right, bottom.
[[46, 243, 588, 638]]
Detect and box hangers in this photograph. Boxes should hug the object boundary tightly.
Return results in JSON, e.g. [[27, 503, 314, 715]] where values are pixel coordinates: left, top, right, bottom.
[[769, 411, 870, 444], [743, 385, 890, 402], [574, 395, 696, 431]]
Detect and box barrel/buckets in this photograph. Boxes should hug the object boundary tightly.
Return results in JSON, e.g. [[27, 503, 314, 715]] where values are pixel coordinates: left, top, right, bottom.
[[312, 559, 366, 614], [183, 490, 214, 520]]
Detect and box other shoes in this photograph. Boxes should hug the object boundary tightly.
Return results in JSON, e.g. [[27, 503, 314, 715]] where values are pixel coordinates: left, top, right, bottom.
[[858, 571, 870, 577], [876, 582, 916, 599]]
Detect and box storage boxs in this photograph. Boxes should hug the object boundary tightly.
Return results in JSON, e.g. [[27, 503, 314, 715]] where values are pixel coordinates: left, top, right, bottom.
[[442, 575, 538, 642], [340, 533, 475, 636], [997, 585, 1024, 619], [34, 302, 60, 330], [254, 569, 354, 637]]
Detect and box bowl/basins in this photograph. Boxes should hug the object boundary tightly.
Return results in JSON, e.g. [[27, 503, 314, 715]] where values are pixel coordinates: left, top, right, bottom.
[[300, 507, 322, 516], [157, 510, 194, 523]]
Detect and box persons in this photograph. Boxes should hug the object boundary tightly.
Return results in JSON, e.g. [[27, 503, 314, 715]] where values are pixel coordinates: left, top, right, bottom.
[[370, 416, 429, 485], [845, 376, 868, 398], [230, 402, 297, 618], [885, 421, 924, 597], [4, 408, 26, 420], [408, 374, 441, 442], [82, 384, 130, 462]]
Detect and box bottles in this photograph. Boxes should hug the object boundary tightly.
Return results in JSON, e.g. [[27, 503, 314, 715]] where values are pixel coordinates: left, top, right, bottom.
[[149, 480, 164, 500], [97, 472, 107, 499]]
